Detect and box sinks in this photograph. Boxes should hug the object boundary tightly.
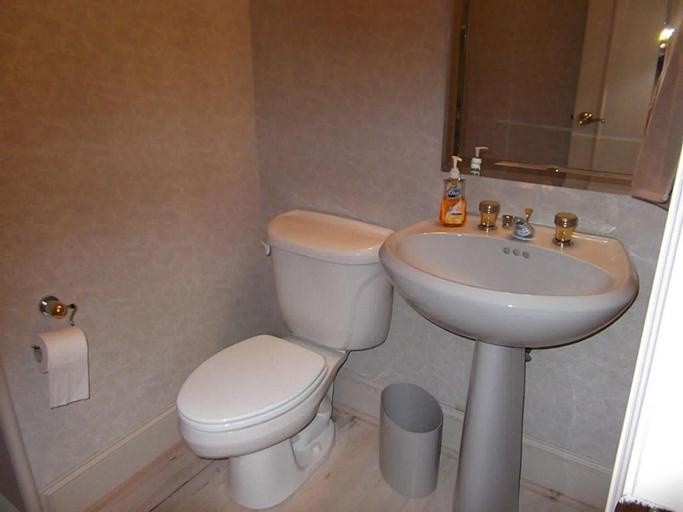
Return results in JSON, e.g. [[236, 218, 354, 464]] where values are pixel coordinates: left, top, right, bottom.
[[376, 210, 642, 351]]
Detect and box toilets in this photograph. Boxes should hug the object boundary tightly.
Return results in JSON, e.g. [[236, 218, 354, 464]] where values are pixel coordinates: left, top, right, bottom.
[[168, 201, 395, 511]]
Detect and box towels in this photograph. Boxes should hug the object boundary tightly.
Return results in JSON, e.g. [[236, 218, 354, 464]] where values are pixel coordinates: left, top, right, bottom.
[[630, 19, 683, 212]]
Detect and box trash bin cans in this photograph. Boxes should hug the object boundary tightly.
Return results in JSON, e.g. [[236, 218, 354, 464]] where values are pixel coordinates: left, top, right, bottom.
[[378, 383, 443, 498]]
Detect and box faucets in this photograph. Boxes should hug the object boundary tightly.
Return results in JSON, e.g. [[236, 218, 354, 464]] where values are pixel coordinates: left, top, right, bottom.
[[502, 205, 537, 240]]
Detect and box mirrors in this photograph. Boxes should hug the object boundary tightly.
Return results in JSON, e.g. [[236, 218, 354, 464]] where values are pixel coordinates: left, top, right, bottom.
[[440, 1, 683, 197]]
